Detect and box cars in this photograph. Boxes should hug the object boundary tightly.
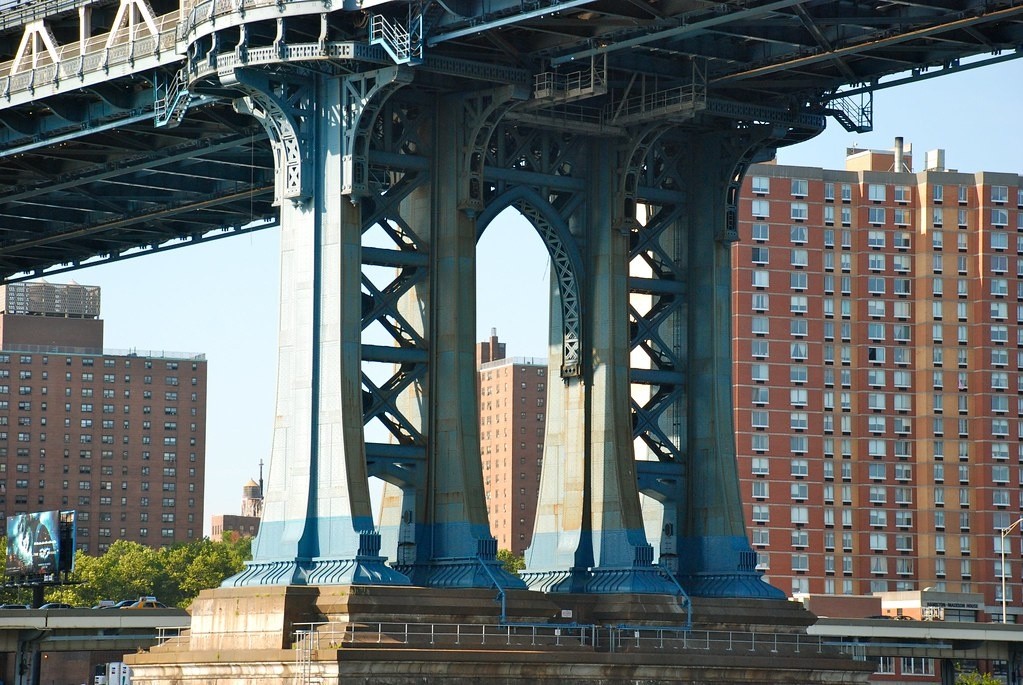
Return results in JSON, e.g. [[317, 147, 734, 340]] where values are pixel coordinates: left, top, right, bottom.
[[894, 615, 917, 621], [37, 602, 75, 610], [119, 596, 177, 609], [102, 599, 138, 610], [91, 604, 114, 609]]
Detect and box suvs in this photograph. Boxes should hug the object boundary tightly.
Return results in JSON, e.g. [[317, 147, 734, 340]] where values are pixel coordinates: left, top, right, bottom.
[[0, 603, 30, 609]]
